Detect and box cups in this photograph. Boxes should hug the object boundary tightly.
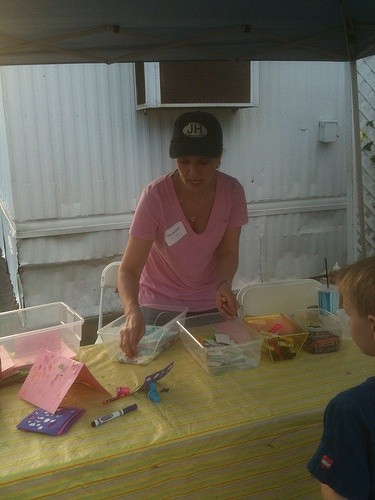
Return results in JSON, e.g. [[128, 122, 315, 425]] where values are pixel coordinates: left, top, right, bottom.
[[317, 284, 340, 319]]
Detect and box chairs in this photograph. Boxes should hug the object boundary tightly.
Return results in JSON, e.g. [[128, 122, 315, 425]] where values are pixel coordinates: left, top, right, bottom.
[[94, 261, 121, 344], [237, 279, 324, 318]]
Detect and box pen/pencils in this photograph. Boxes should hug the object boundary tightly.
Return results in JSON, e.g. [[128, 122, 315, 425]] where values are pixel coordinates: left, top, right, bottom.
[[103, 396, 120, 404]]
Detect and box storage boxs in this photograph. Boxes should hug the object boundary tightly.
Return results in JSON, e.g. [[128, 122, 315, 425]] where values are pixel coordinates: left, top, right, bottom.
[[0, 302, 352, 387]]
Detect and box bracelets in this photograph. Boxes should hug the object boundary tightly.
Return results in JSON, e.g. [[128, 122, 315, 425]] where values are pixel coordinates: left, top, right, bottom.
[[217, 280, 232, 291]]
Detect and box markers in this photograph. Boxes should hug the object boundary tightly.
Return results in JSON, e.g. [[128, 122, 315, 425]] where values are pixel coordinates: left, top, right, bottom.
[[91, 403, 138, 427], [269, 324, 281, 334]]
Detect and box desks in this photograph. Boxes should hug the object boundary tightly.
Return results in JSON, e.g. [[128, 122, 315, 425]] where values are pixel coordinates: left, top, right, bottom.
[[0, 310, 375, 500]]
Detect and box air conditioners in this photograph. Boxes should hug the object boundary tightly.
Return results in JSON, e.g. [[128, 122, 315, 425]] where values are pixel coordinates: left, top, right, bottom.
[[133, 62, 260, 111]]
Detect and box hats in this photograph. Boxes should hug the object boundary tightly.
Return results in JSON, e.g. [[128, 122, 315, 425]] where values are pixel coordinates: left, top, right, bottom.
[[168, 111, 222, 157]]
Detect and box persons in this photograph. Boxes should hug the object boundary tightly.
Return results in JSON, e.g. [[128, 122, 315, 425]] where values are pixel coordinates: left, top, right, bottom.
[[306, 255, 375, 500], [118, 111, 248, 358]]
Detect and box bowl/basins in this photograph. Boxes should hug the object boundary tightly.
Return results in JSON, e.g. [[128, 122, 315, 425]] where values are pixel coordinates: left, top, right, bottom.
[[244, 314, 307, 361], [286, 308, 345, 355], [176, 312, 264, 375], [0, 302, 84, 387], [97, 303, 189, 365]]
